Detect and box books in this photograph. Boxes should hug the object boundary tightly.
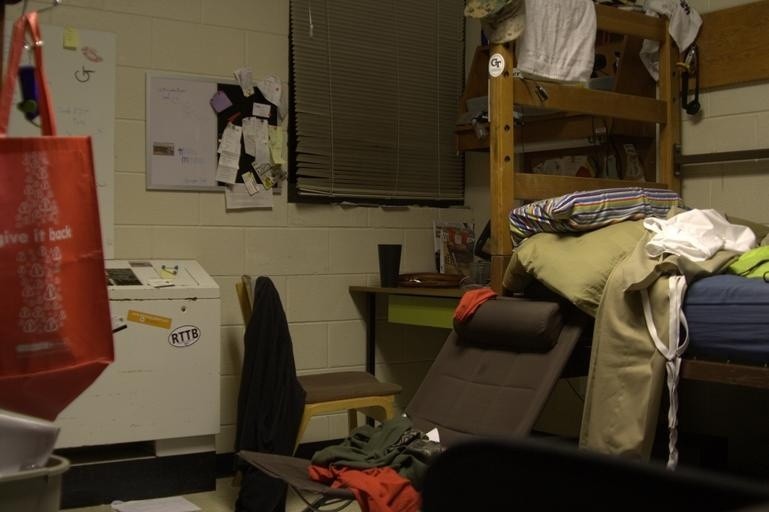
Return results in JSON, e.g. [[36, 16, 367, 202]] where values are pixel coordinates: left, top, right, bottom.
[[431, 219, 479, 276]]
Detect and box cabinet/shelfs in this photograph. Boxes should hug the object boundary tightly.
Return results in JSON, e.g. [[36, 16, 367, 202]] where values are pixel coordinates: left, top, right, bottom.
[[455, 0, 685, 153]]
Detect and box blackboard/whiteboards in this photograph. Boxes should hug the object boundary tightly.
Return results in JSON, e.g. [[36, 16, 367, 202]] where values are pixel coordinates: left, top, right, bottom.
[[146, 71, 283, 194]]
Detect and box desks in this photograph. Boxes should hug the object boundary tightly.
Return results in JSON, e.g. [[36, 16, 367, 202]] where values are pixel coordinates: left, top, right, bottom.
[[348, 255, 495, 443]]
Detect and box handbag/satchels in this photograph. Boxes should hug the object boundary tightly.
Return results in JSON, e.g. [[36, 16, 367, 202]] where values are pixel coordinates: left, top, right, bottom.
[[0, 137, 115, 415]]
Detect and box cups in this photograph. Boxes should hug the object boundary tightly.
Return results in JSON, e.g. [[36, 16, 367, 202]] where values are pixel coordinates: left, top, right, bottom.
[[377, 244, 402, 287]]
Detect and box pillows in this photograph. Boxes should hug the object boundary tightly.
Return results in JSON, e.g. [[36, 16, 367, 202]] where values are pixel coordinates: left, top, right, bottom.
[[504, 187, 679, 242]]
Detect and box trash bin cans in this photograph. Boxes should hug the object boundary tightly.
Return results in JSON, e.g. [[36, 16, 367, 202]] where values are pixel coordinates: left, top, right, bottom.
[[0, 454, 71, 511]]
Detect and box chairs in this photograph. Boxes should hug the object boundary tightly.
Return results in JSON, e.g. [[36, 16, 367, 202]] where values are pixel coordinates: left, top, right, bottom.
[[239, 273, 403, 455], [426, 443, 768, 512], [231, 286, 598, 508]]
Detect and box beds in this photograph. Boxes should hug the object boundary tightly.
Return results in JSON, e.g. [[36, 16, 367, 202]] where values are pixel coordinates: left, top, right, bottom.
[[496, 163, 767, 486]]
